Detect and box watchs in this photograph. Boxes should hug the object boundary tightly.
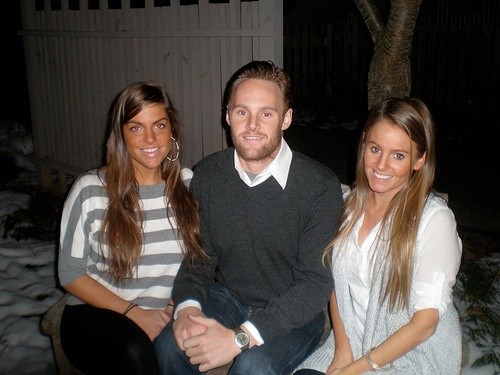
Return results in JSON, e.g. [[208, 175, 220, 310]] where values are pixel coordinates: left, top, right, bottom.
[[233, 327, 250, 352]]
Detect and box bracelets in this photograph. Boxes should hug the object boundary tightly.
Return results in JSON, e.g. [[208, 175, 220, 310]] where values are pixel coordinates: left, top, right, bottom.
[[366, 348, 392, 371], [123, 302, 138, 316], [168, 303, 174, 306]]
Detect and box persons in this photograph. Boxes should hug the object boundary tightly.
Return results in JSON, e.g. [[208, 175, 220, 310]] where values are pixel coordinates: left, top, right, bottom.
[[57, 82, 193, 375], [293, 98, 462, 375], [153, 61, 345, 375]]
